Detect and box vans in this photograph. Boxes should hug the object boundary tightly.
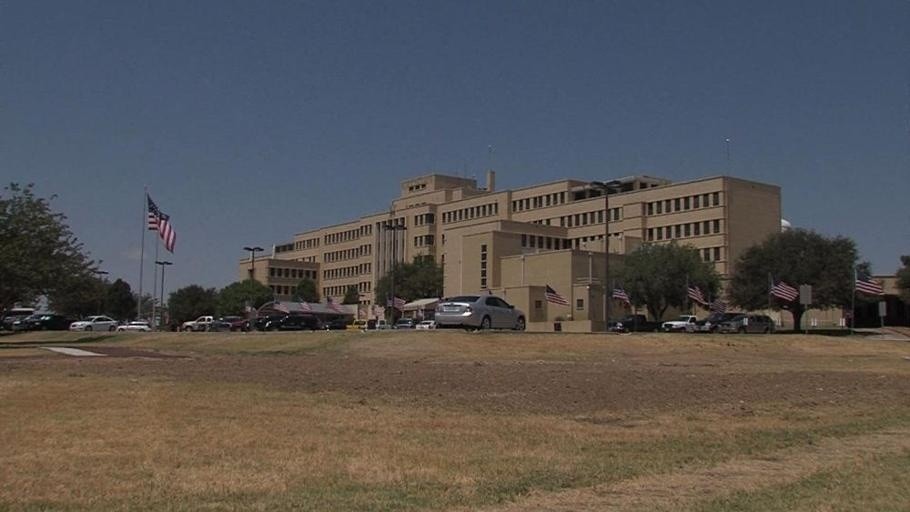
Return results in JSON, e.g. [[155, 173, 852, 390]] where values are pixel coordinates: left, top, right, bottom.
[[719, 313, 776, 335]]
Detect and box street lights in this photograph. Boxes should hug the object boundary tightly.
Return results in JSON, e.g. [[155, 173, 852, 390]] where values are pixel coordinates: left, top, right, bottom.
[[588, 180, 624, 331], [154, 260, 173, 331], [92, 271, 108, 315], [240, 246, 264, 329], [382, 224, 407, 329]]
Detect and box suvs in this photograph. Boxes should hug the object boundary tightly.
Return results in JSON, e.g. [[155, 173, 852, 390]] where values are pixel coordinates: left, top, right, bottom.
[[692, 312, 745, 333], [118, 321, 152, 332]]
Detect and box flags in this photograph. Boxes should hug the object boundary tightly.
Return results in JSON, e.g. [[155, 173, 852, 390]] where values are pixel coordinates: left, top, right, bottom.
[[546, 285, 571, 306], [613, 287, 632, 307], [146, 192, 177, 255], [855, 269, 884, 295], [328, 297, 344, 313], [300, 297, 312, 309], [275, 298, 290, 314], [388, 295, 406, 309], [688, 277, 708, 305], [770, 275, 799, 303]]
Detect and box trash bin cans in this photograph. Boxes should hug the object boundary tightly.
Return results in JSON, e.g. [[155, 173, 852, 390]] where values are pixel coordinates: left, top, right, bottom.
[[554, 322, 562, 331], [367, 319, 376, 330]]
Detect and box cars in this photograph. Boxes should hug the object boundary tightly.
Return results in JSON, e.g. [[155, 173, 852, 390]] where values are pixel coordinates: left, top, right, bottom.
[[251, 313, 437, 331], [1, 307, 74, 331], [434, 294, 526, 331], [69, 314, 119, 332]]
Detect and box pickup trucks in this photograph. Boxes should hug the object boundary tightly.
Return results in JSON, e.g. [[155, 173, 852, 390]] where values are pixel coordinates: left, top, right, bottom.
[[607, 314, 663, 332], [661, 315, 712, 332], [181, 315, 233, 332]]
